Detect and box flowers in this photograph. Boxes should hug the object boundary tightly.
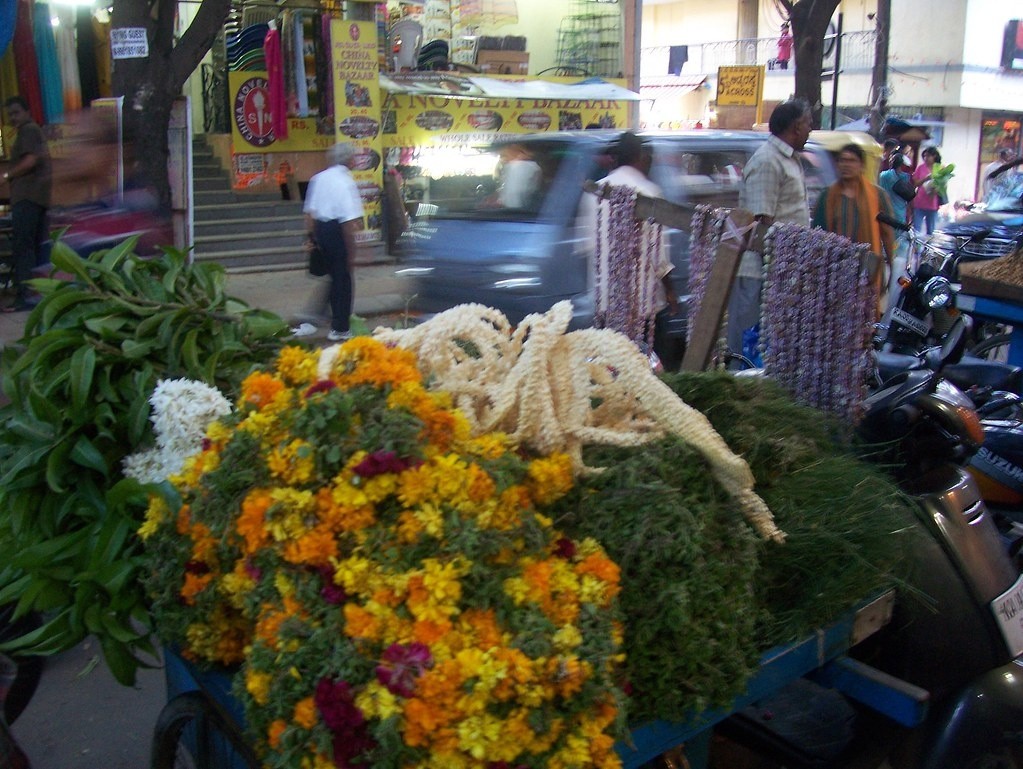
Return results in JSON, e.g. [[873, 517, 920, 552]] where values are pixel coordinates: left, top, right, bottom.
[[120, 336, 628, 768]]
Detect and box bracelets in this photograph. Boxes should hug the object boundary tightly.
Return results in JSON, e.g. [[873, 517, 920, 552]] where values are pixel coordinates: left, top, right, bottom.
[[3, 171, 10, 181]]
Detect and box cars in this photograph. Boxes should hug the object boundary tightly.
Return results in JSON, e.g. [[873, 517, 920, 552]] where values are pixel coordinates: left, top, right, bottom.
[[925, 156, 1023, 278]]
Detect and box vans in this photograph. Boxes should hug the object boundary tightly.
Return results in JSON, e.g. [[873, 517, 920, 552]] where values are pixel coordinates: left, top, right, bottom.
[[401, 131, 837, 355]]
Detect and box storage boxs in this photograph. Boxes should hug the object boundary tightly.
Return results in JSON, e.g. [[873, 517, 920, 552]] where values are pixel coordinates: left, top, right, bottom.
[[476, 49, 530, 74]]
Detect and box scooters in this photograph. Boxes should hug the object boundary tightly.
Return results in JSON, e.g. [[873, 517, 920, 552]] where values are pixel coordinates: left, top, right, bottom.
[[720, 212, 1023, 702]]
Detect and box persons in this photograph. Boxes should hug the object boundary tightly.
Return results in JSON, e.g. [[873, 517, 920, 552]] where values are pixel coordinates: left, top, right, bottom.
[[730, 102, 811, 357], [811, 145, 896, 292], [983, 149, 1009, 197], [879, 138, 948, 253], [1, 95, 54, 314], [777, 22, 794, 70], [303, 145, 365, 344], [577, 129, 678, 363]]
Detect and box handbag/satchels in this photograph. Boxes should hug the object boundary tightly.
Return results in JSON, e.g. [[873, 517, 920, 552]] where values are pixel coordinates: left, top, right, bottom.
[[309, 248, 328, 277], [892, 179, 916, 201]]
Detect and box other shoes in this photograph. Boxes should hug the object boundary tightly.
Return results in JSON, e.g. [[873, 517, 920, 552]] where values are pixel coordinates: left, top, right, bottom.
[[289, 322, 318, 337], [2, 296, 38, 312], [327, 329, 355, 341]]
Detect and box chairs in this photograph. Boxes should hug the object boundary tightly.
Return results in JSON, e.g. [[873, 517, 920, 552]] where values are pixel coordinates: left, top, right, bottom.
[[222, 0, 315, 72], [387, 20, 449, 73]]
